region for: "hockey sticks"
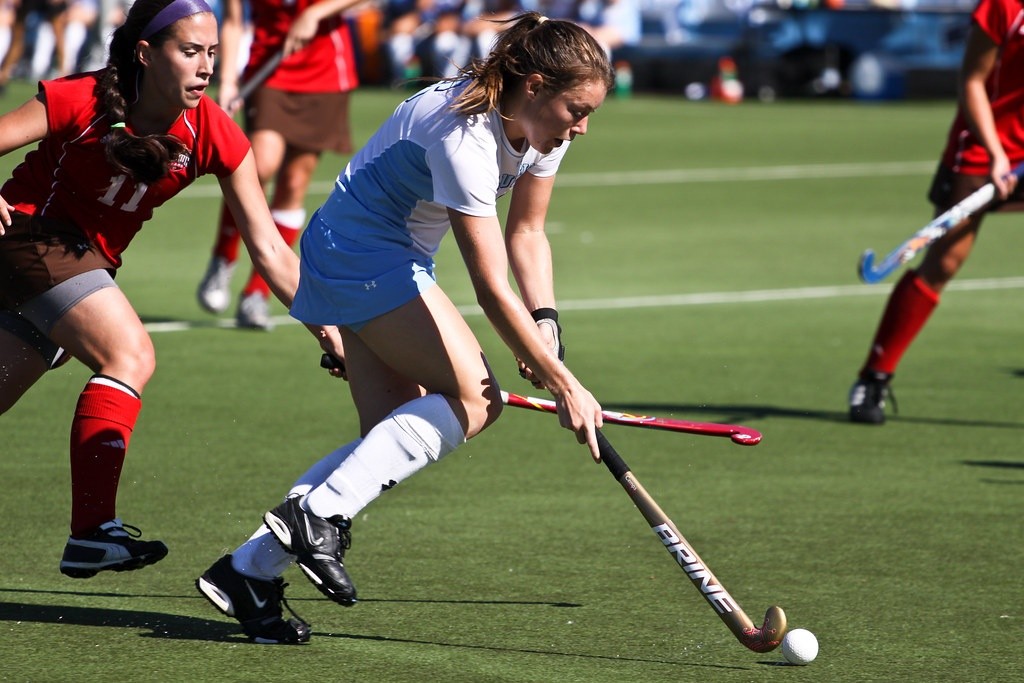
[854,162,1024,287]
[225,50,286,111]
[593,427,789,656]
[317,351,765,448]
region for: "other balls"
[779,627,820,666]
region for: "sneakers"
[193,555,309,644]
[848,368,899,425]
[59,519,167,578]
[261,494,358,607]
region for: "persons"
[197,2,366,331]
[195,11,616,647]
[0,1,346,581]
[0,0,905,106]
[850,0,1023,427]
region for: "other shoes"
[198,252,229,312]
[237,292,270,329]
